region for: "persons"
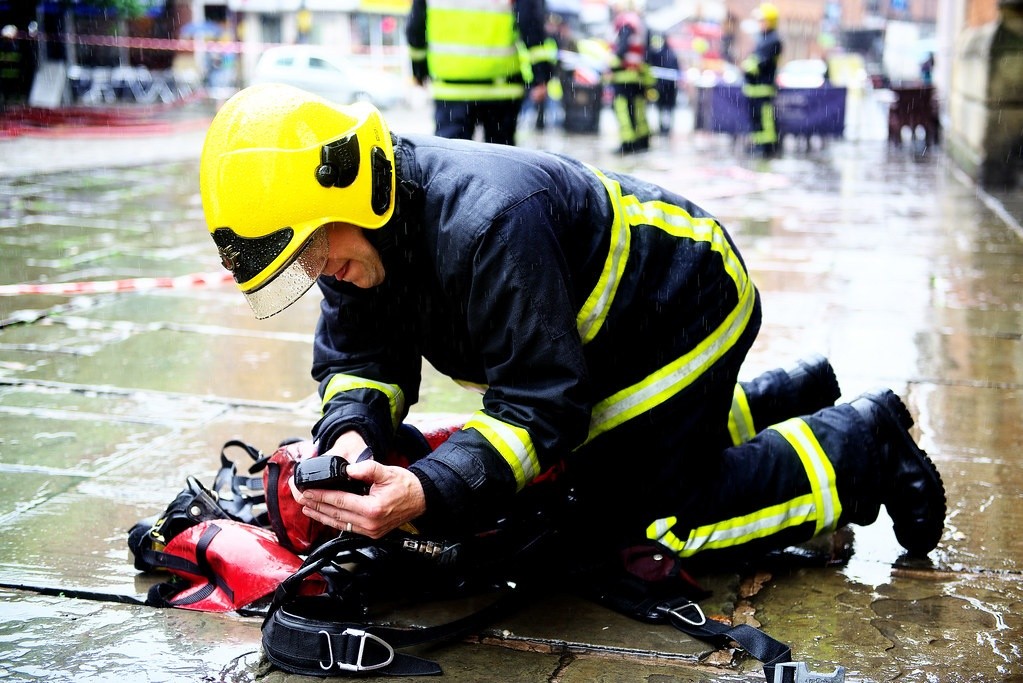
[406,0,556,147]
[531,1,680,151]
[201,83,947,581]
[743,6,782,155]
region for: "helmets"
[198,80,396,295]
[753,1,780,31]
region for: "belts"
[262,603,482,675]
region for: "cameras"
[292,455,372,498]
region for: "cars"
[696,57,848,136]
[250,47,405,112]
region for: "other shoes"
[617,137,648,153]
[746,141,779,157]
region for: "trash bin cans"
[565,81,603,136]
[693,86,765,134]
[775,84,848,151]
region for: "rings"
[347,523,352,532]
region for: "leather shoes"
[783,352,844,415]
[851,389,946,556]
